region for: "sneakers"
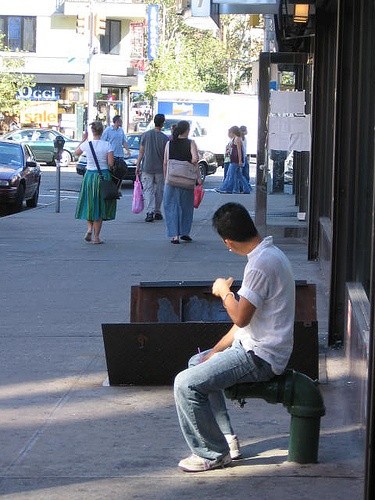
[223,435,242,460]
[177,452,231,472]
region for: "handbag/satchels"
[88,141,118,200]
[132,175,144,214]
[167,140,197,190]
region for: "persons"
[99,116,129,198]
[217,126,250,194]
[174,202,293,471]
[75,122,117,244]
[163,121,198,243]
[136,114,169,222]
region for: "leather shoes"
[144,213,154,222]
[154,213,163,220]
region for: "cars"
[0,139,41,212]
[2,128,80,166]
[76,133,217,186]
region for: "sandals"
[84,232,92,242]
[170,238,180,245]
[180,235,191,242]
[92,241,104,245]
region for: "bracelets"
[222,293,235,309]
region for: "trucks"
[146,90,258,154]
